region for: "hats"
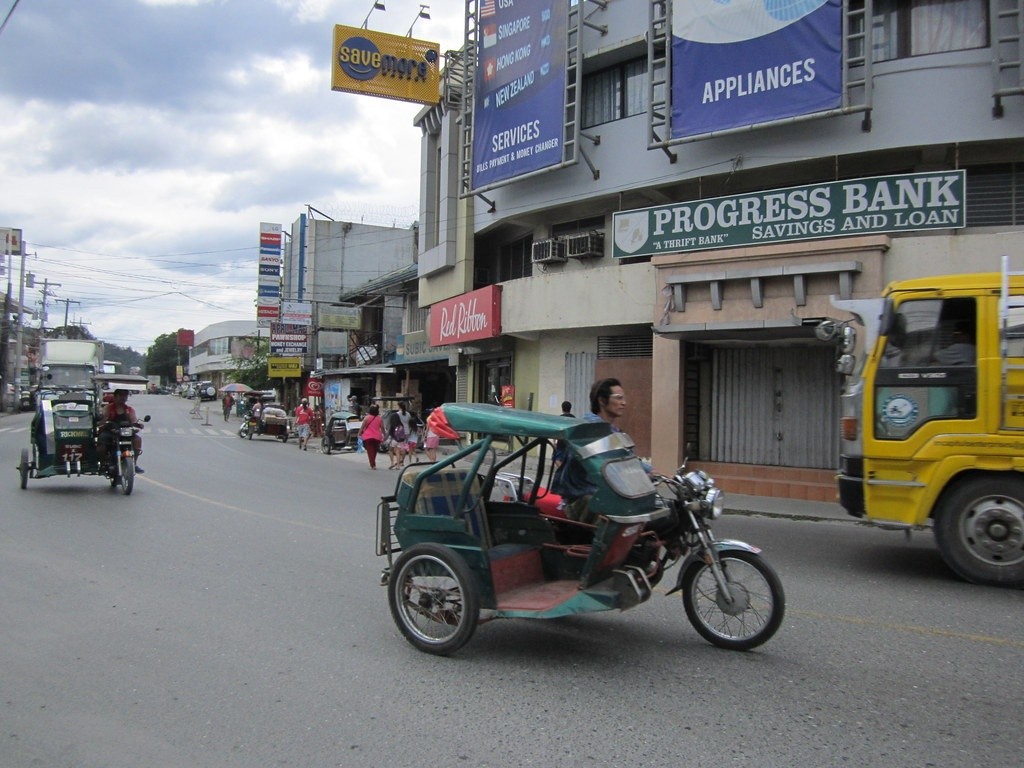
[302,398,308,403]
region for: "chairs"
[403,469,544,594]
[333,426,346,441]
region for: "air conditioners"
[567,231,603,259]
[532,239,567,263]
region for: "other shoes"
[135,465,145,474]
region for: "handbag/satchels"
[394,424,406,441]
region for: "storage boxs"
[264,413,286,425]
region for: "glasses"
[606,393,626,402]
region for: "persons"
[405,418,420,463]
[223,391,234,421]
[396,401,411,465]
[251,399,263,419]
[96,388,144,473]
[425,426,439,462]
[551,376,662,526]
[923,321,976,366]
[294,397,314,451]
[553,401,575,467]
[349,396,361,416]
[359,404,386,470]
[383,412,403,470]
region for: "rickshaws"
[15,387,152,496]
[372,394,429,455]
[237,389,290,444]
[320,412,364,455]
[370,397,786,657]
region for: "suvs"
[193,380,217,402]
[173,381,197,399]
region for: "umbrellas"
[219,383,252,392]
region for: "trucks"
[31,336,105,420]
[797,255,1024,589]
